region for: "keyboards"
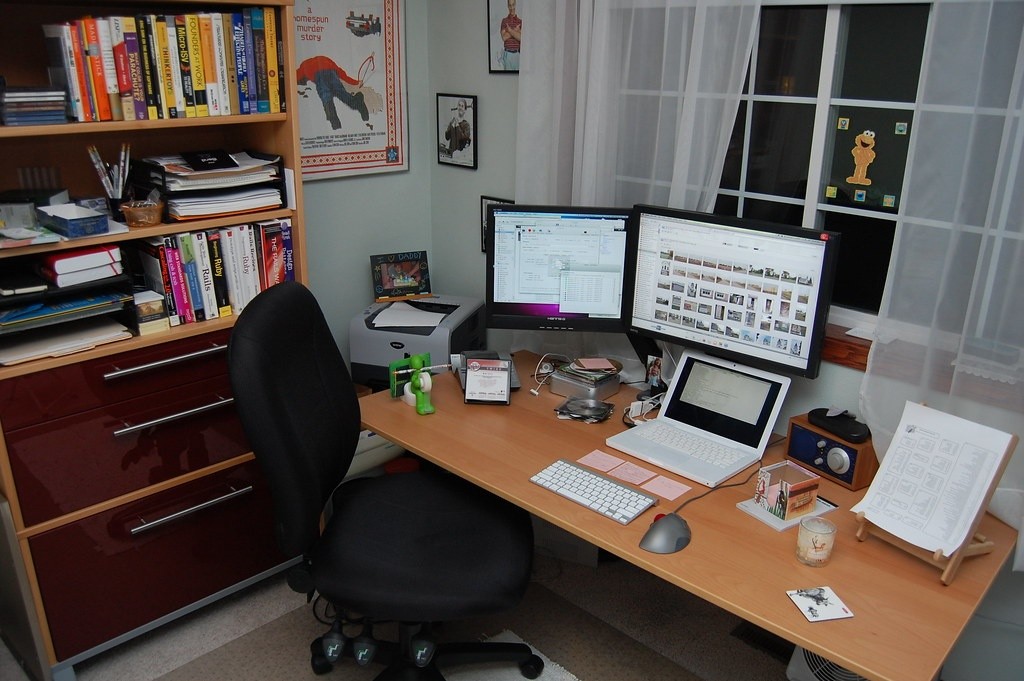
[528,458,659,525]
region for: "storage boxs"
[344,380,406,479]
[755,460,820,521]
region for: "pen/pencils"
[86,141,135,199]
[392,364,454,375]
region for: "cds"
[567,400,607,414]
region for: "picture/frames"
[481,195,516,254]
[486,0,522,74]
[370,250,431,299]
[436,92,478,170]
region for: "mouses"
[639,512,692,553]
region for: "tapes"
[418,373,432,393]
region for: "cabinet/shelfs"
[0,0,308,681]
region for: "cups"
[107,195,131,222]
[795,516,838,567]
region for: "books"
[140,218,295,327]
[0,271,47,295]
[41,244,122,287]
[736,495,839,532]
[0,7,288,125]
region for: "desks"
[357,350,1019,681]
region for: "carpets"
[434,629,581,681]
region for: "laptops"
[606,347,792,488]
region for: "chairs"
[228,280,544,681]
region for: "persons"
[500,0,522,70]
[444,99,470,154]
[390,262,403,278]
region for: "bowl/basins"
[119,200,166,227]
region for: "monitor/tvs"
[630,204,843,380]
[484,203,633,334]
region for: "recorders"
[785,412,880,491]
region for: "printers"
[349,293,487,389]
